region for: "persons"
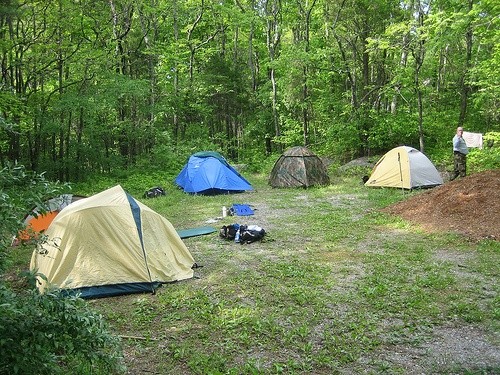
[449,126,468,180]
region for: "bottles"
[222,206,227,217]
[235,229,241,243]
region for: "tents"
[30,185,196,300]
[175,151,254,194]
[12,195,90,248]
[268,147,330,189]
[363,146,444,189]
